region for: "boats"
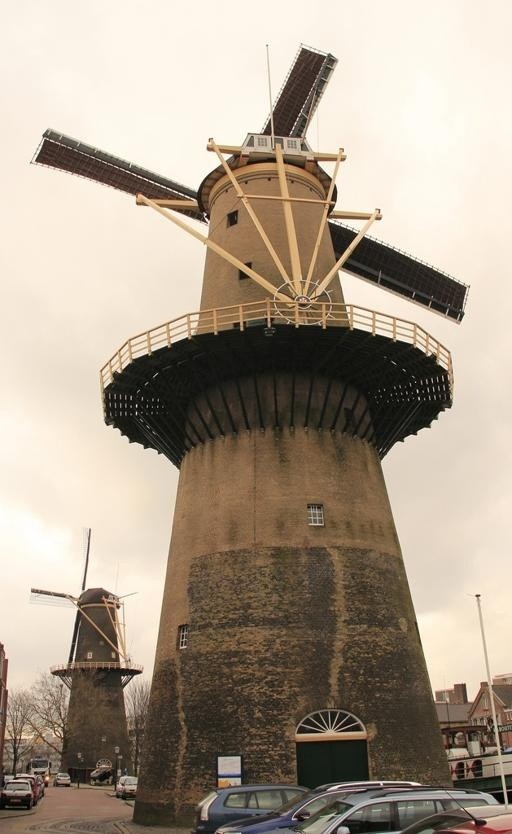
[442,725,512,795]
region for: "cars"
[53,772,71,787]
[0,774,45,810]
[115,775,139,800]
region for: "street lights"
[77,752,82,789]
[114,746,120,788]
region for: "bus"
[26,758,51,787]
